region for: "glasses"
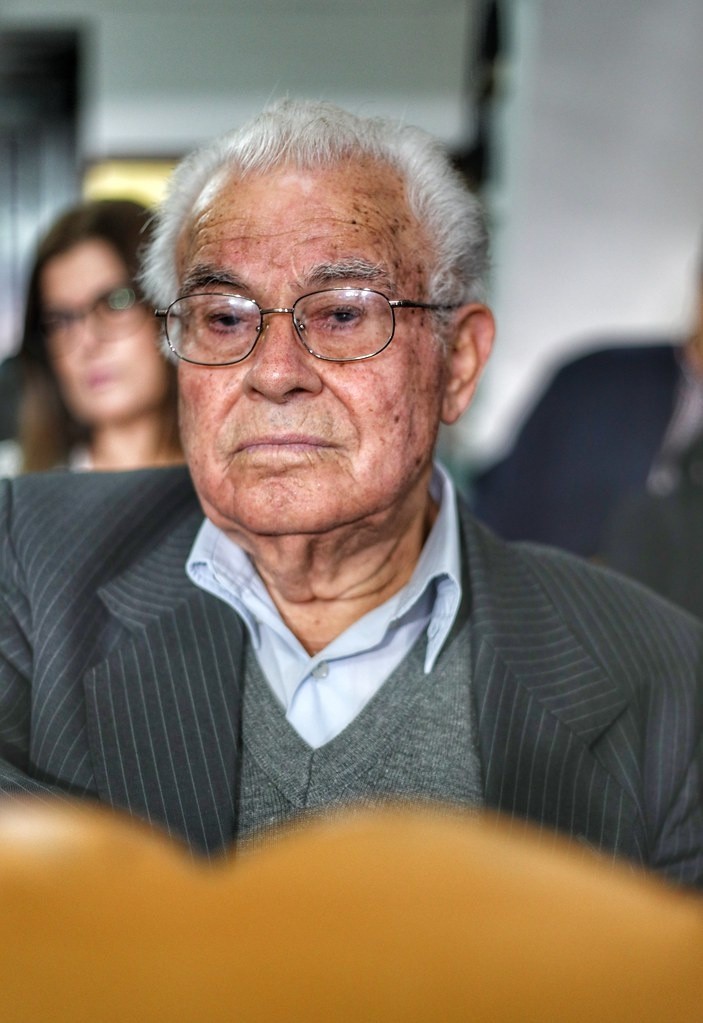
[154,286,461,362]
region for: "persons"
[469,255,702,617]
[0,198,187,476]
[0,90,702,1023]
